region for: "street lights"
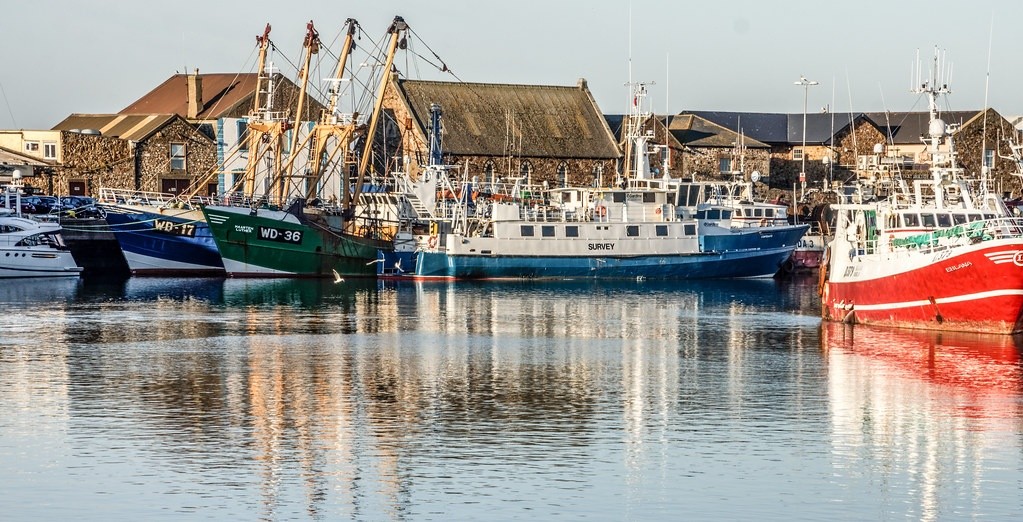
[360,63,382,182]
[794,76,820,203]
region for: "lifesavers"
[357,225,370,237]
[427,236,438,249]
[595,206,607,218]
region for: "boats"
[0,11,813,287]
[815,43,1023,337]
